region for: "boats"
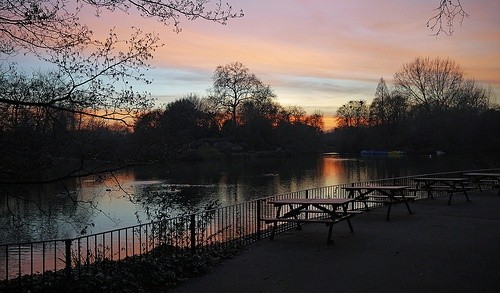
[321,152,340,158]
[360,150,406,157]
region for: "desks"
[341,185,413,221]
[462,172,500,192]
[267,198,356,245]
[410,176,472,205]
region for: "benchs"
[479,179,498,182]
[352,199,398,203]
[258,218,340,223]
[427,185,475,189]
[406,188,456,191]
[364,194,419,199]
[296,209,364,214]
